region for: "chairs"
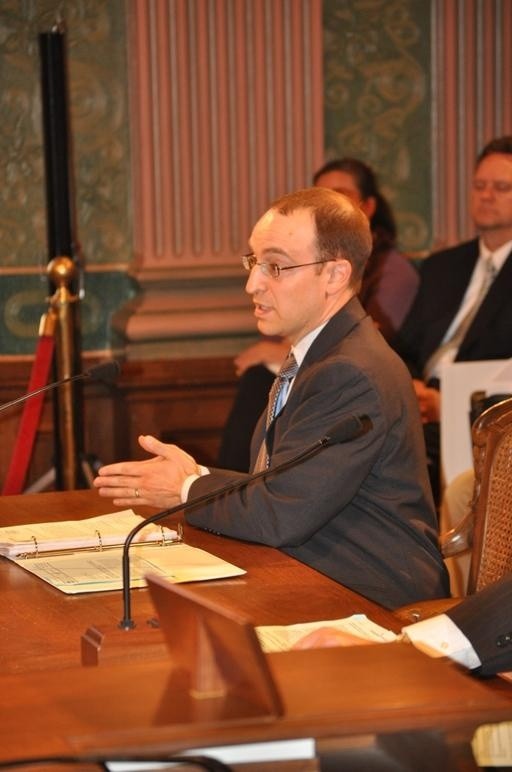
[392,397,512,624]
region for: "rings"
[135,488,139,497]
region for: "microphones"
[0,359,122,413]
[121,411,375,627]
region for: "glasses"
[242,252,337,278]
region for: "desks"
[0,487,512,771]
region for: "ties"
[423,254,498,385]
[266,353,299,469]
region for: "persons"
[392,135,512,498]
[292,565,511,680]
[216,159,422,473]
[94,188,448,611]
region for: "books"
[1,508,247,595]
